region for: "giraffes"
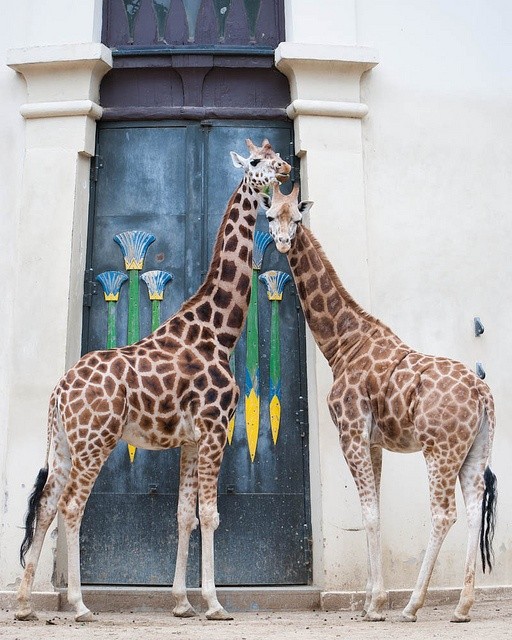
[258,181,497,623]
[14,138,292,622]
